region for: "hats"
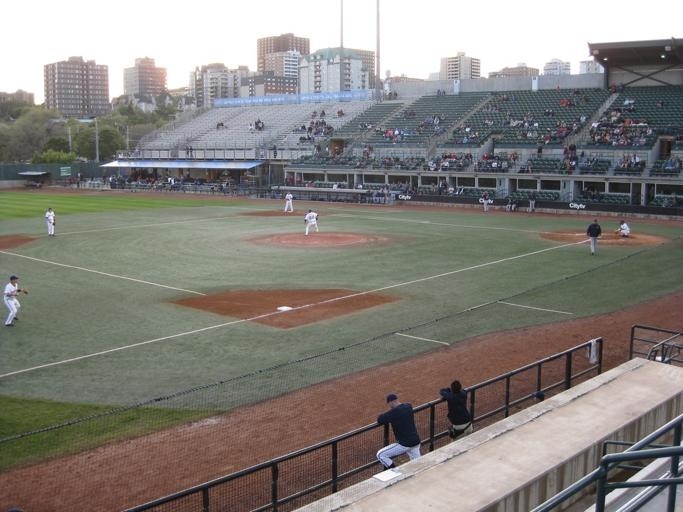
[386,394,397,402]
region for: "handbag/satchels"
[448,427,464,438]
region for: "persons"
[64,146,216,194]
[386,81,683,214]
[216,107,386,205]
[615,220,631,238]
[45,207,57,237]
[2,275,28,327]
[375,392,421,472]
[439,379,475,443]
[586,218,602,256]
[283,191,296,212]
[304,209,319,236]
[531,391,545,404]
